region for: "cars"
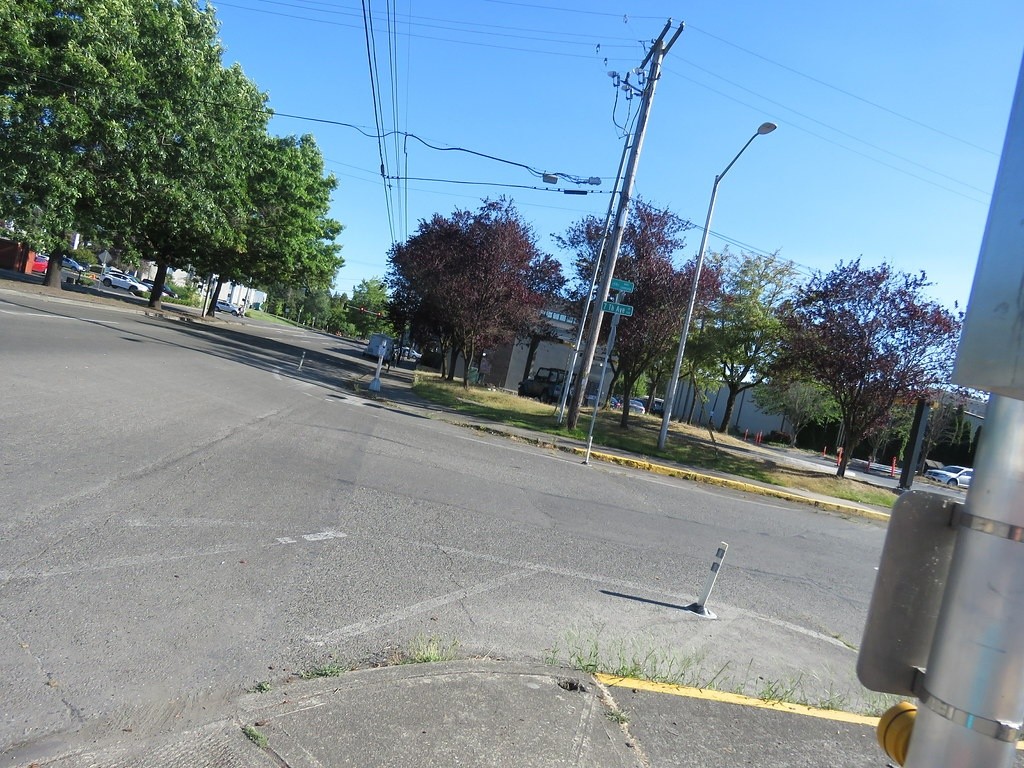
[215,301,240,317]
[608,396,666,413]
[61,257,84,272]
[31,256,49,274]
[398,347,423,360]
[99,269,179,300]
[923,465,974,488]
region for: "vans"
[362,333,396,363]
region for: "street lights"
[654,122,779,449]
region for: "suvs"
[518,368,589,407]
